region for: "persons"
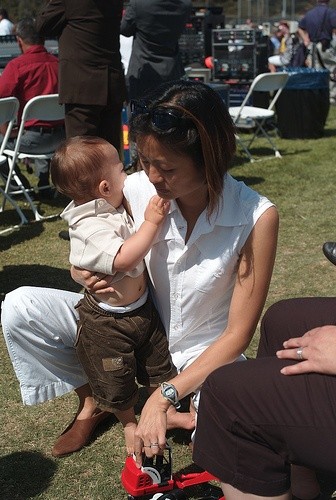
[297,0,336,104]
[0,18,68,202]
[1,81,280,458]
[192,297,336,500]
[0,9,17,35]
[268,23,293,73]
[120,0,191,161]
[49,135,196,455]
[35,0,128,160]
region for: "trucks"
[121,440,226,500]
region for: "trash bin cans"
[274,68,330,140]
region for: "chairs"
[228,72,288,163]
[0,97,28,235]
[0,94,65,222]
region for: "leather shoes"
[322,241,336,267]
[52,411,114,456]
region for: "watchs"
[160,382,181,409]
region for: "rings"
[297,347,303,359]
[151,443,158,446]
[143,446,151,447]
[95,273,101,280]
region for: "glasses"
[131,98,185,130]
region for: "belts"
[22,124,62,134]
